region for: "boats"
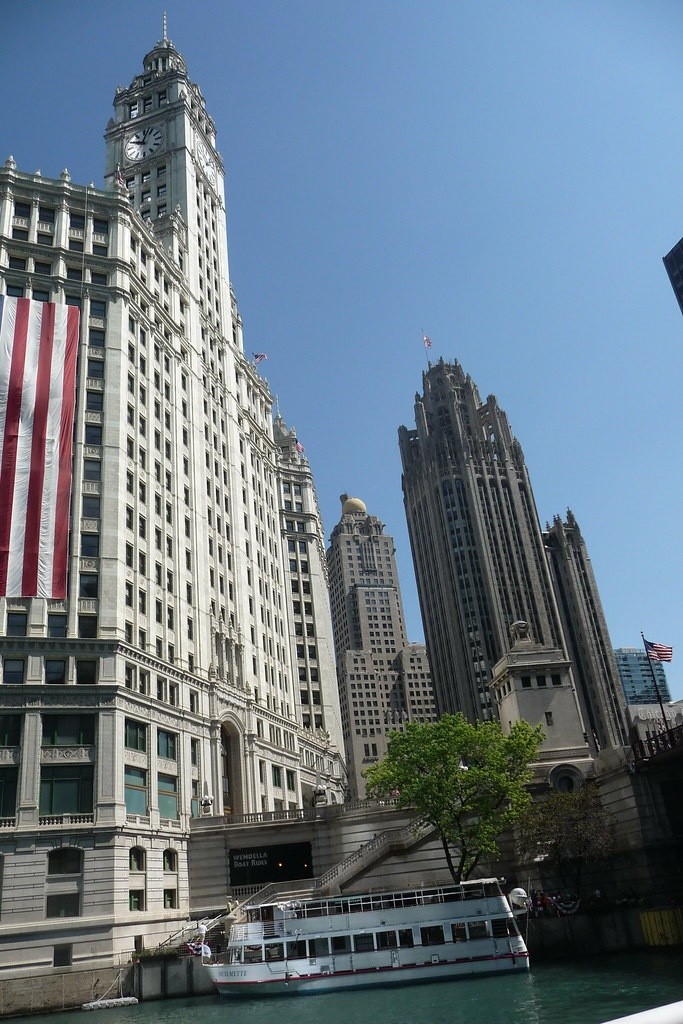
[196,876,533,998]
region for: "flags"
[644,638,673,664]
[0,297,85,604]
[295,438,305,454]
[424,334,432,350]
[253,352,268,365]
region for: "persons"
[226,899,232,914]
[528,886,642,919]
[234,897,239,908]
[392,785,400,796]
[198,922,210,944]
